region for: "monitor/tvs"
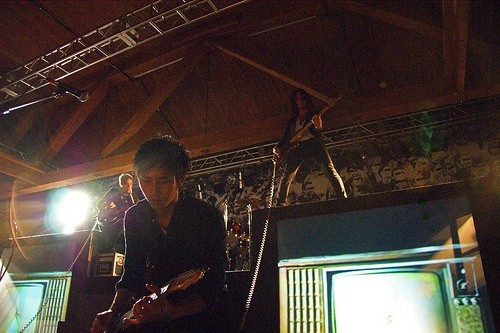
[245,177,499,332]
[9,271,73,333]
[276,244,484,333]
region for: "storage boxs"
[95,252,125,277]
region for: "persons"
[88,134,238,333]
[271,87,350,208]
[96,173,137,253]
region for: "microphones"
[96,187,114,208]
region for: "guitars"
[96,198,135,227]
[99,264,216,333]
[273,94,342,164]
